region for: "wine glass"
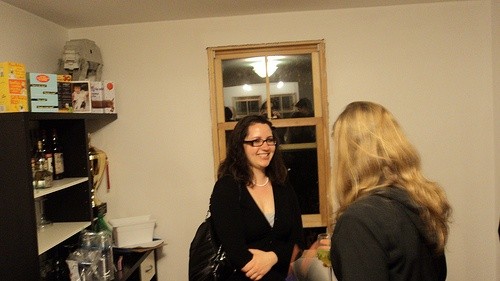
[316,233,333,281]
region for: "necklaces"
[252,176,269,187]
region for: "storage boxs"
[0,61,28,113]
[113,221,155,246]
[90,80,116,113]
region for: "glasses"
[243,138,277,147]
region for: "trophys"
[88,132,110,220]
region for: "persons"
[224,97,319,215]
[72,83,89,110]
[207,115,305,281]
[189,217,315,281]
[316,101,453,281]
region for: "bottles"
[36,140,47,181]
[46,138,53,180]
[94,217,113,245]
[49,134,66,180]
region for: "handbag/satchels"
[188,171,242,281]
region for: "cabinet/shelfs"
[0,112,158,281]
